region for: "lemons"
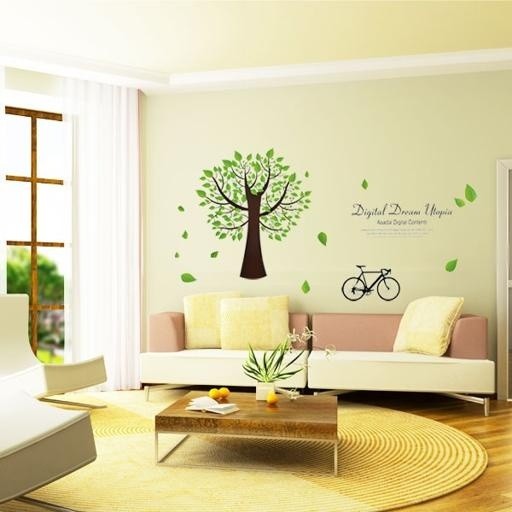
[267,392,278,405]
[208,386,230,400]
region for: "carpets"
[14,390,488,512]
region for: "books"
[184,396,241,416]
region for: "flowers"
[243,326,338,400]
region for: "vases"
[256,382,278,401]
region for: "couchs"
[308,313,496,416]
[140,312,310,401]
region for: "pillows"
[185,292,251,350]
[220,295,293,350]
[393,296,464,356]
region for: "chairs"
[1,293,107,408]
[0,387,97,504]
